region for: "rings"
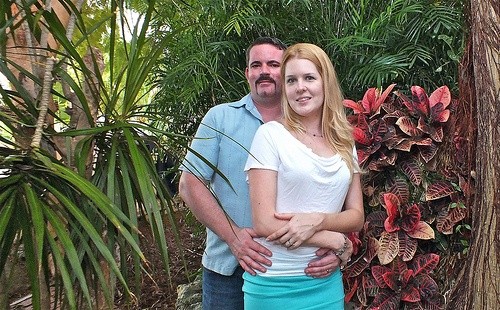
[287,239,292,246]
[327,270,330,274]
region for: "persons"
[242,43,364,310]
[178,37,341,310]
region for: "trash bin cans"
[156,162,176,199]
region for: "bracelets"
[335,233,350,257]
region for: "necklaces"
[310,131,324,138]
